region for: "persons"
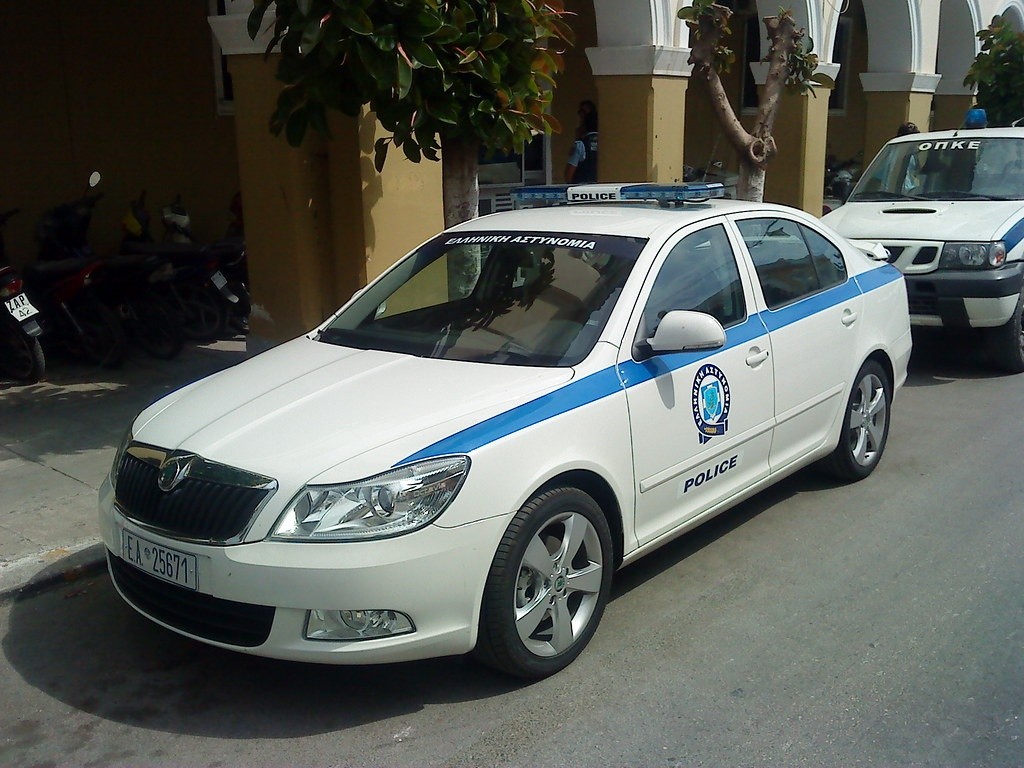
[563,101,599,184]
[885,122,923,196]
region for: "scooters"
[0,171,253,384]
[823,151,861,203]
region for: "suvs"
[818,110,1024,375]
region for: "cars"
[98,182,914,682]
